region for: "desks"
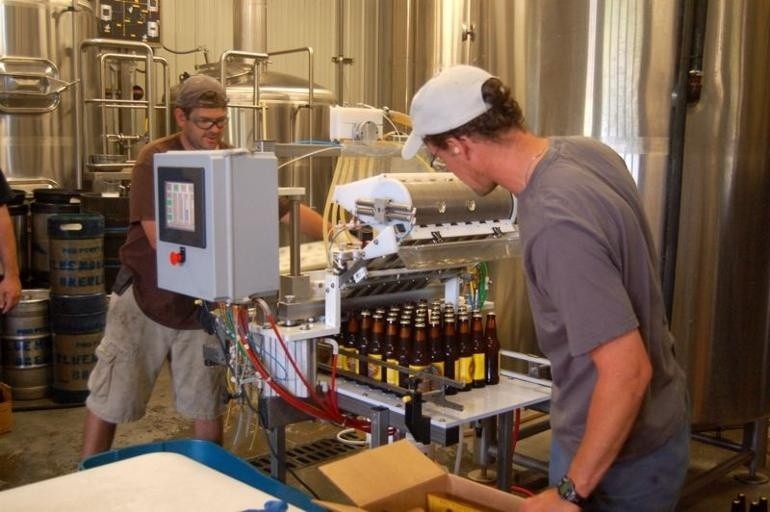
[0,449,310,512]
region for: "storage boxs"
[311,439,526,512]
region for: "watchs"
[556,475,586,508]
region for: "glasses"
[429,139,451,170]
[185,116,229,130]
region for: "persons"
[81,74,334,461]
[0,168,22,317]
[400,64,692,511]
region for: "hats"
[401,65,502,159]
[170,75,229,108]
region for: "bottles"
[319,296,501,403]
